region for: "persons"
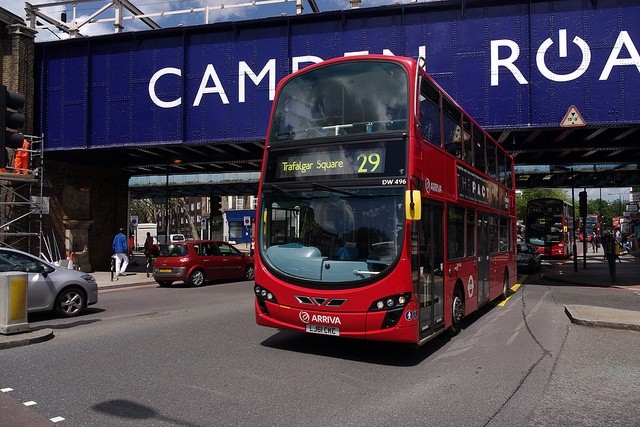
[603,230,618,282]
[113,228,130,277]
[590,232,600,252]
[144,232,153,257]
[14,132,30,176]
[620,234,627,246]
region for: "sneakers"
[116,273,121,276]
[119,271,126,276]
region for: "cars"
[0,247,97,316]
[517,244,541,270]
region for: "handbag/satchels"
[596,242,600,248]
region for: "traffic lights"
[0,85,25,168]
[210,196,222,216]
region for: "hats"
[120,228,125,230]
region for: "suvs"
[153,240,254,286]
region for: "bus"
[579,214,598,241]
[525,198,574,257]
[254,54,518,346]
